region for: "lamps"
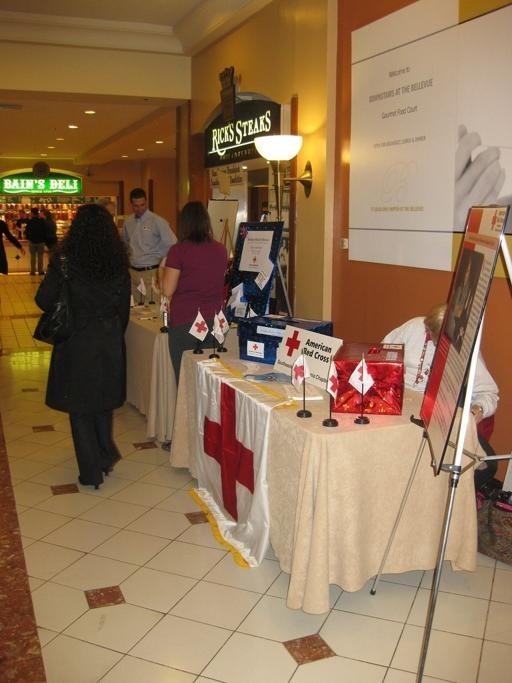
[253,134,315,223]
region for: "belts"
[130,264,158,271]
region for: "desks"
[172,347,478,614]
[129,304,238,443]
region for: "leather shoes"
[78,455,115,489]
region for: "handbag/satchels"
[32,302,74,345]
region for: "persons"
[33,203,131,488]
[157,201,227,451]
[122,188,177,305]
[16,207,59,275]
[378,303,500,494]
[454,125,512,232]
[0,216,26,274]
